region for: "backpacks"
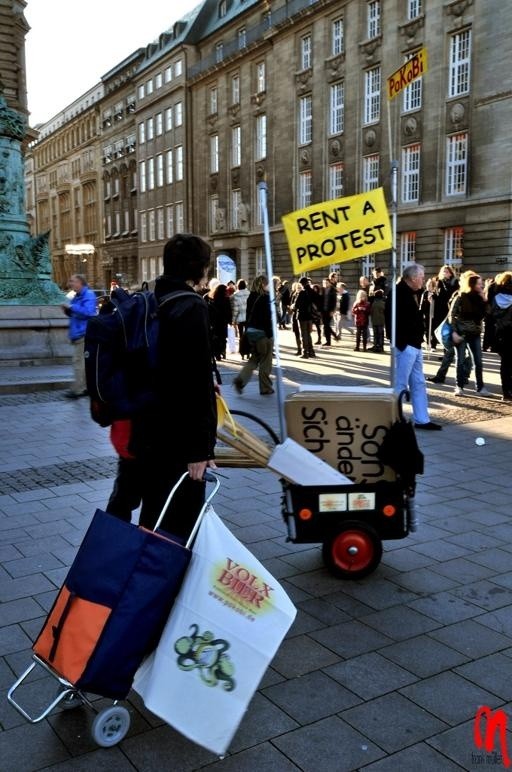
[84,280,205,427]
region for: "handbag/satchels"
[434,317,455,350]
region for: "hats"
[227,280,235,286]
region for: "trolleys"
[216,159,410,581]
[6,467,220,750]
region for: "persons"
[105,234,218,540]
[61,273,97,398]
[196,263,512,431]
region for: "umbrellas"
[376,389,424,475]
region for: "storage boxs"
[284,388,399,485]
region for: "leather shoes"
[426,376,444,383]
[295,348,315,358]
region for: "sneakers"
[60,389,88,400]
[414,422,442,430]
[315,336,340,345]
[454,386,463,396]
[260,389,274,395]
[242,355,250,360]
[475,387,492,396]
[354,345,383,352]
[233,377,244,394]
[216,355,226,360]
